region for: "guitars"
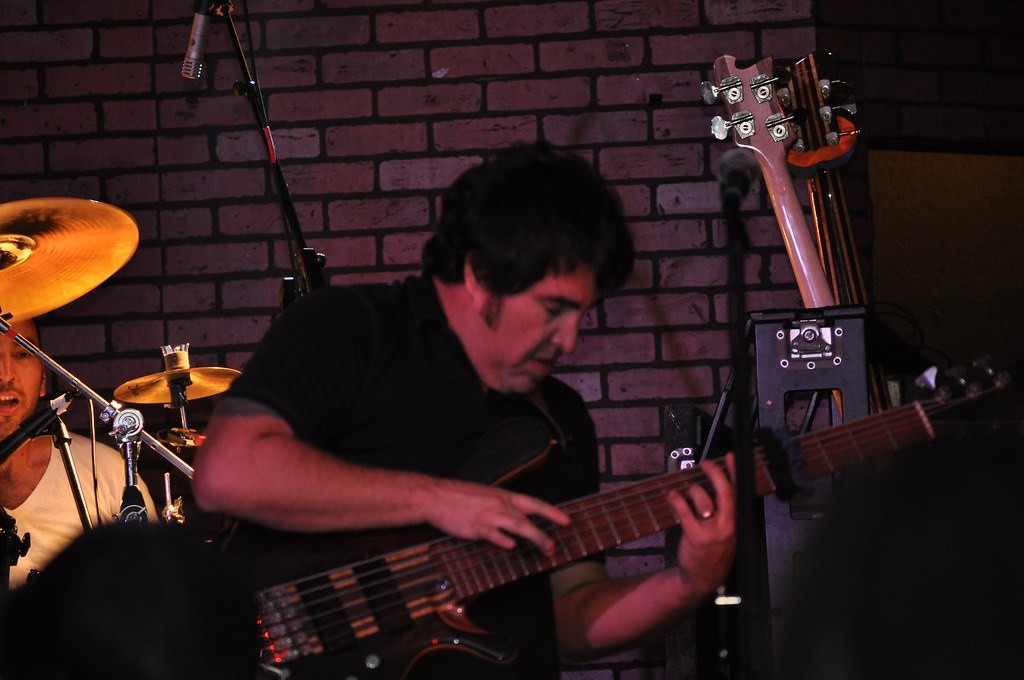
[208,379,1024,680]
[699,49,864,309]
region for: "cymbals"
[0,192,145,333]
[112,364,247,409]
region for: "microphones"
[0,391,75,466]
[717,149,759,203]
[181,0,214,80]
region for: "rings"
[701,511,712,520]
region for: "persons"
[191,137,738,680]
[0,316,163,593]
